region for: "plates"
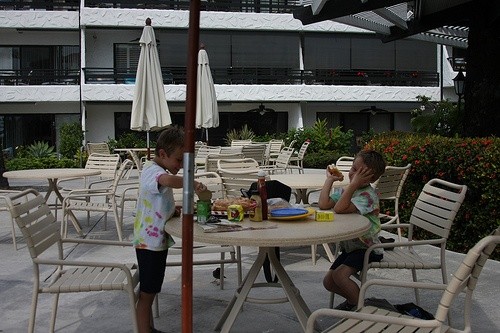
[270,208,308,216]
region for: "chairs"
[0,139,500,333]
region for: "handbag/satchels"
[240,180,292,203]
[395,303,434,320]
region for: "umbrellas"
[130,25,172,160]
[196,49,219,141]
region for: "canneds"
[196,200,211,224]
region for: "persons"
[320,151,386,312]
[134,129,207,333]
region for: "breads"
[328,164,344,180]
[212,197,249,211]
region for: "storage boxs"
[315,210,334,222]
[197,200,211,223]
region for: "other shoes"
[334,299,358,311]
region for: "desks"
[113,148,156,152]
[165,204,372,333]
[3,169,101,233]
[270,174,349,263]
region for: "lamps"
[452,69,466,95]
[259,111,265,115]
[371,110,376,115]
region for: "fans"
[360,106,386,111]
[247,102,275,112]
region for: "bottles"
[249,190,262,222]
[258,171,268,221]
